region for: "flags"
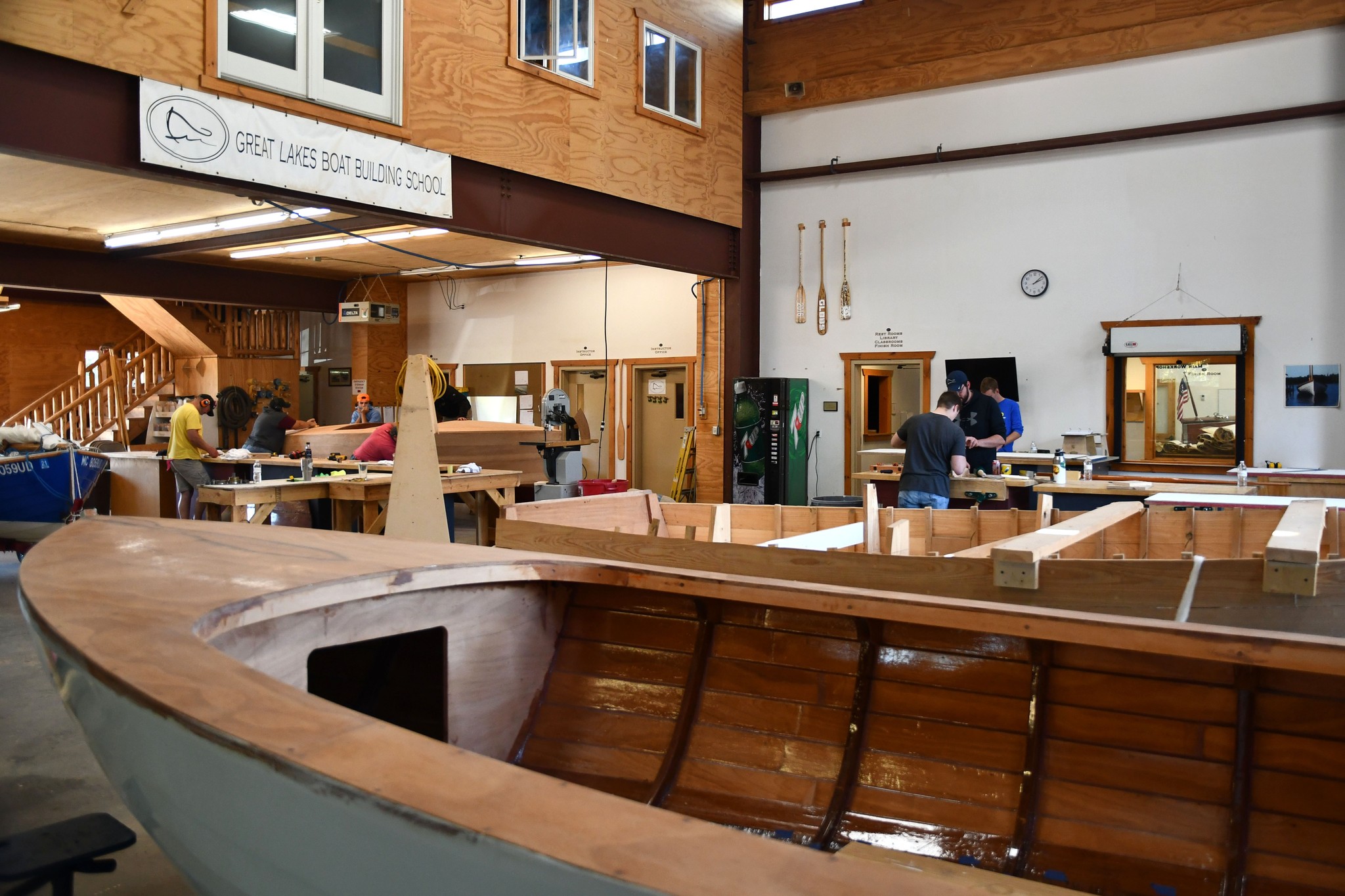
[1176,376,1189,423]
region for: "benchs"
[990,501,1145,591]
[755,521,865,552]
[1260,499,1327,598]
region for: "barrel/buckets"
[810,495,863,508]
[578,479,629,497]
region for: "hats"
[197,394,215,416]
[357,393,370,402]
[945,370,968,391]
[270,397,291,408]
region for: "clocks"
[1021,270,1048,297]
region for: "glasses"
[956,383,966,393]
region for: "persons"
[430,376,473,423]
[167,394,218,520]
[350,393,382,424]
[350,422,399,462]
[891,370,1024,509]
[242,398,319,456]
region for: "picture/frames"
[328,367,352,387]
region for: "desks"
[99,450,523,548]
[850,452,1345,511]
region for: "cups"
[1001,464,1011,475]
[447,465,453,474]
[301,458,313,481]
[358,464,368,479]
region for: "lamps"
[398,253,602,275]
[104,204,331,249]
[229,227,449,259]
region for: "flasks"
[1052,448,1066,484]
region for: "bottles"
[305,442,312,459]
[1083,457,1093,482]
[253,459,262,484]
[1030,440,1037,454]
[734,381,765,480]
[1237,460,1247,488]
